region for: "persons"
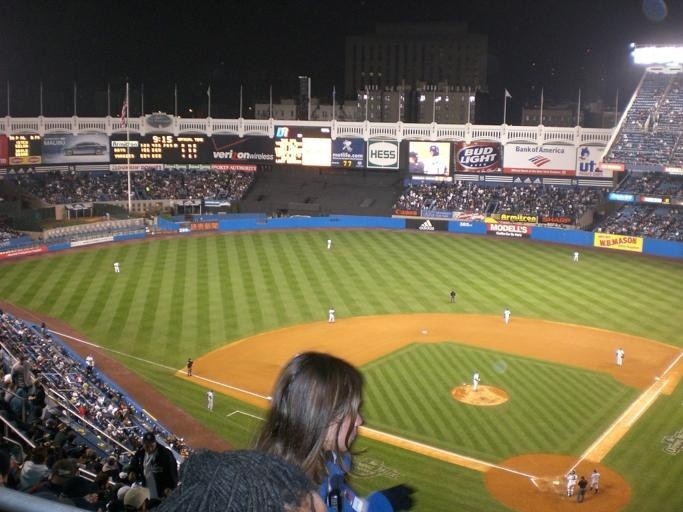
[206,389,215,413]
[503,308,510,324]
[450,291,455,303]
[0,308,196,511]
[247,352,415,511]
[577,476,588,503]
[157,447,331,511]
[473,369,482,392]
[393,180,599,227]
[572,250,579,264]
[566,470,577,496]
[588,468,600,494]
[616,347,624,366]
[9,170,258,208]
[327,239,331,249]
[595,174,683,242]
[113,261,119,272]
[327,306,336,323]
[608,73,683,169]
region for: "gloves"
[380,483,416,511]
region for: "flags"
[120,94,128,129]
[505,89,511,97]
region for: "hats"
[123,486,150,509]
[117,486,130,499]
[143,432,156,444]
